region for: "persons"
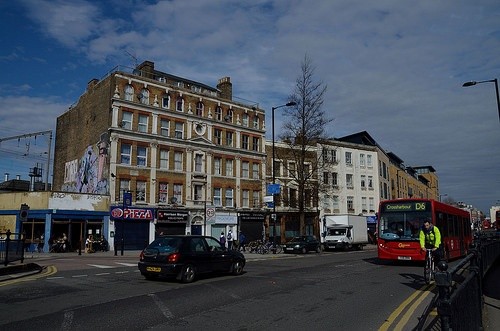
[420,221,442,275]
[39,233,108,253]
[396,225,404,236]
[220,230,246,253]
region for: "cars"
[473,234,487,243]
[283,235,322,254]
[138,236,246,284]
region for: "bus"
[378,198,472,261]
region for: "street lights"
[462,78,500,122]
[270,102,296,254]
[396,169,412,198]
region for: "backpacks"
[227,232,232,240]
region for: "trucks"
[323,214,367,251]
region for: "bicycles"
[256,239,282,255]
[419,249,439,284]
